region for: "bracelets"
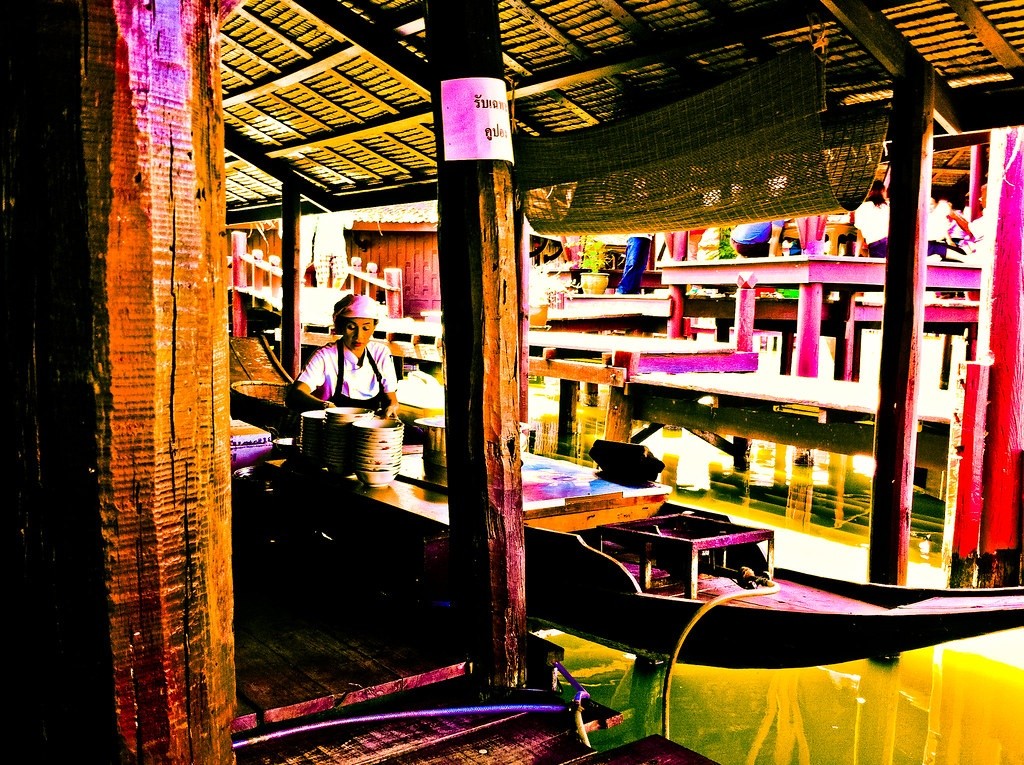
[323,401,334,409]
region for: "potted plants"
[565,234,610,296]
[528,258,583,327]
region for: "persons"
[730,220,784,258]
[615,233,655,295]
[284,295,399,421]
[854,178,988,301]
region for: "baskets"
[230,381,305,438]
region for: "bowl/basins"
[273,438,294,452]
[300,408,405,488]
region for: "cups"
[580,273,610,295]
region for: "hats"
[333,294,380,334]
